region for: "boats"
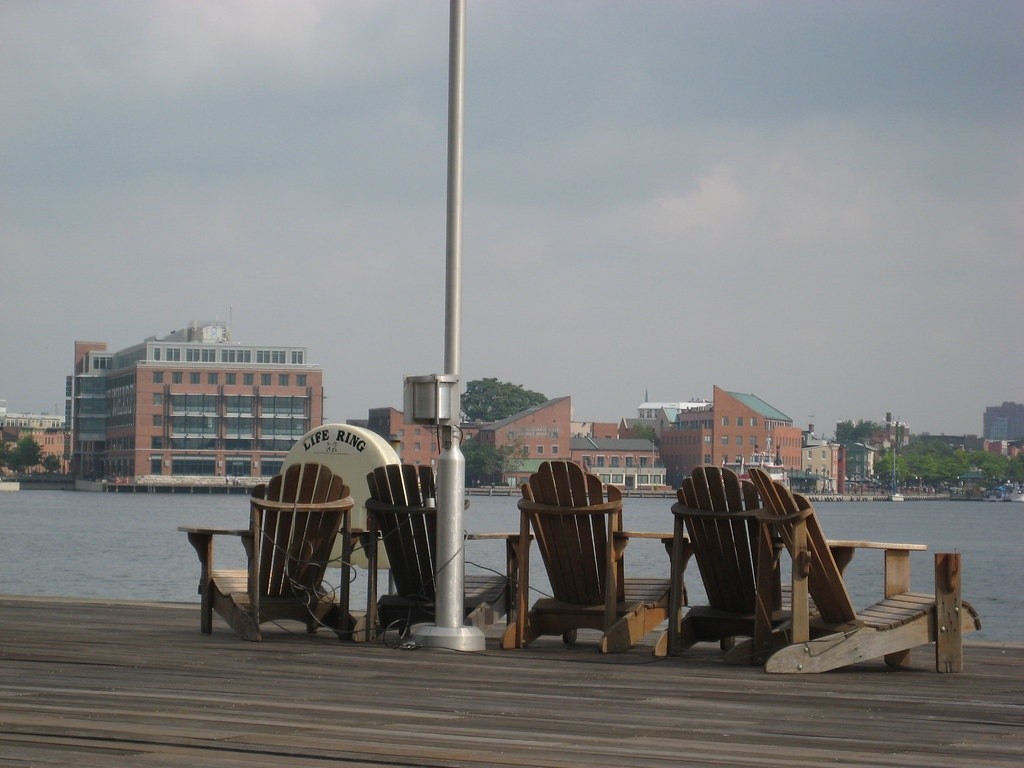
[891,494,904,502]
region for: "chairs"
[651,465,786,671]
[500,460,694,654]
[177,463,357,642]
[351,464,535,642]
[723,467,984,674]
